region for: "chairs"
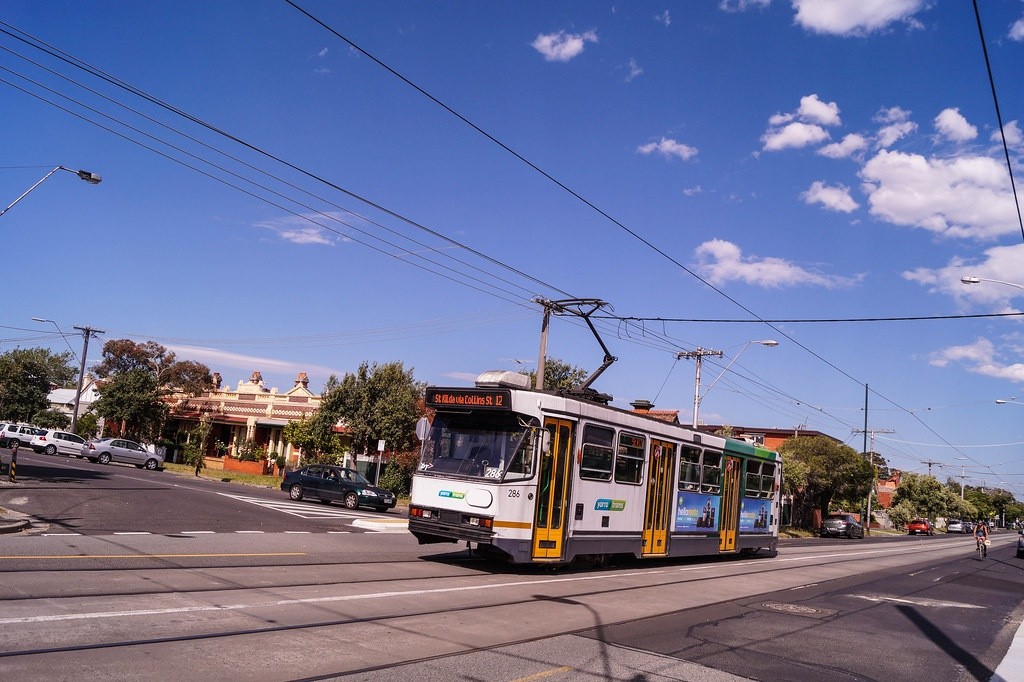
[469,447,491,462]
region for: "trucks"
[1006,518,1024,529]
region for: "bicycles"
[975,535,987,560]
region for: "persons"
[327,470,335,480]
[974,520,988,556]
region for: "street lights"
[31,317,83,435]
[693,340,779,431]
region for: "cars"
[280,465,398,512]
[964,522,974,533]
[1017,528,1024,557]
[81,438,163,471]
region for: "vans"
[29,429,86,458]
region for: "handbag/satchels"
[984,540,991,545]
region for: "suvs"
[948,520,965,533]
[821,514,864,539]
[0,422,38,449]
[909,519,933,536]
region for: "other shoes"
[976,548,979,550]
[984,555,987,559]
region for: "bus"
[407,369,785,572]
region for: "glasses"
[978,523,981,524]
